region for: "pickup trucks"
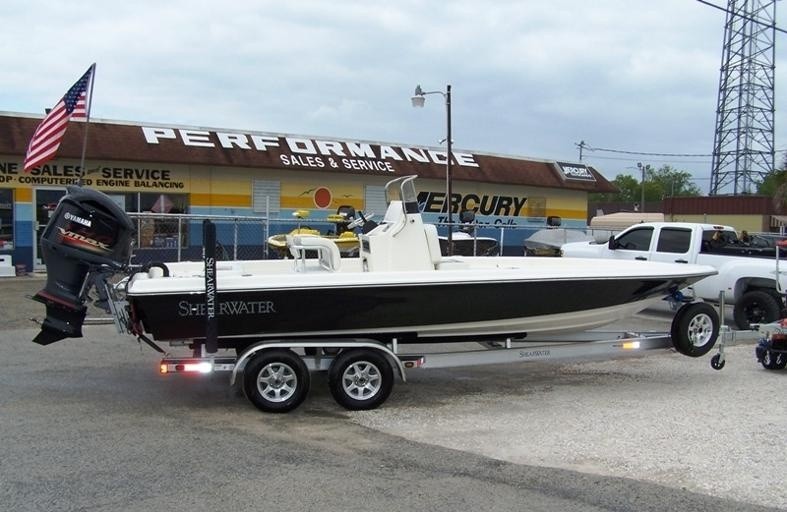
[559,222,786,331]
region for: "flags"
[22,63,94,173]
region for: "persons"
[708,230,728,254]
[740,230,749,247]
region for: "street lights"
[410,83,455,256]
[637,162,650,212]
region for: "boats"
[521,215,597,257]
[437,210,499,256]
[266,206,367,258]
[29,174,717,337]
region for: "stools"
[291,234,341,272]
